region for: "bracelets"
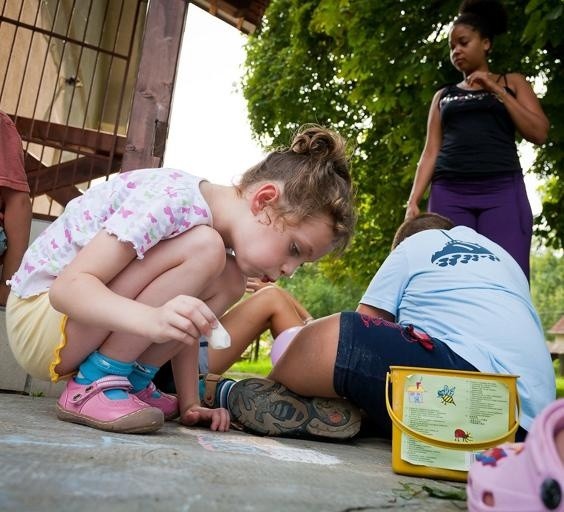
[494,88,507,103]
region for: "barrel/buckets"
[383,365,521,481]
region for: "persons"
[1,110,34,309]
[217,214,559,444]
[4,123,355,434]
[199,270,317,375]
[404,13,552,293]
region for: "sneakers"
[54,374,180,434]
[224,377,364,440]
